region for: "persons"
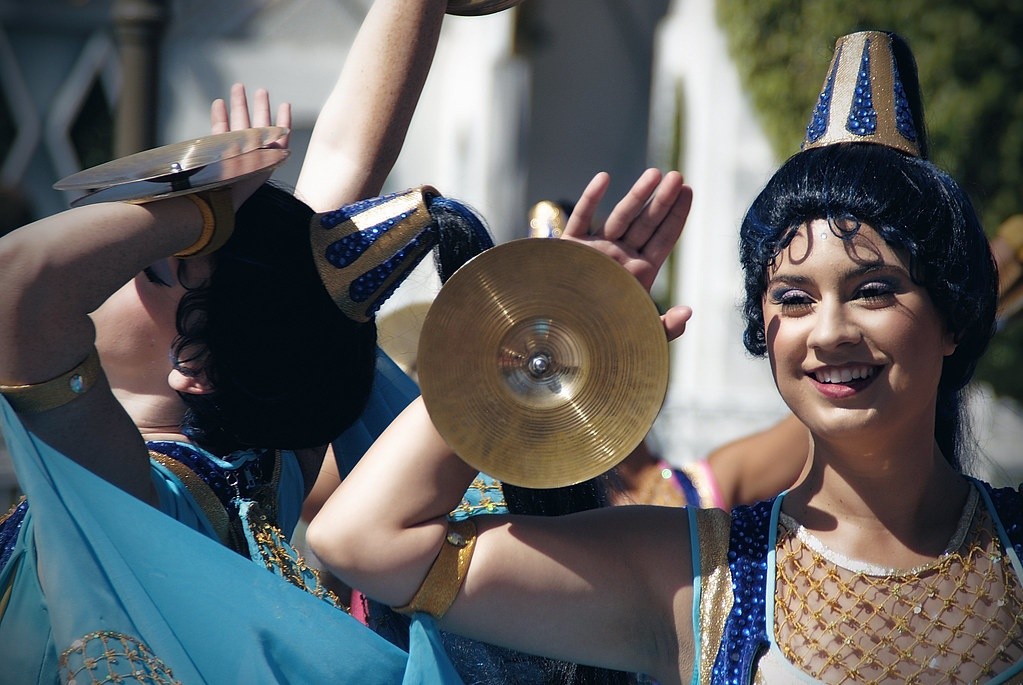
[0,0,808,683]
[304,143,1023,685]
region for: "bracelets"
[173,193,216,257]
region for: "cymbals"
[51,123,291,212]
[415,231,673,494]
